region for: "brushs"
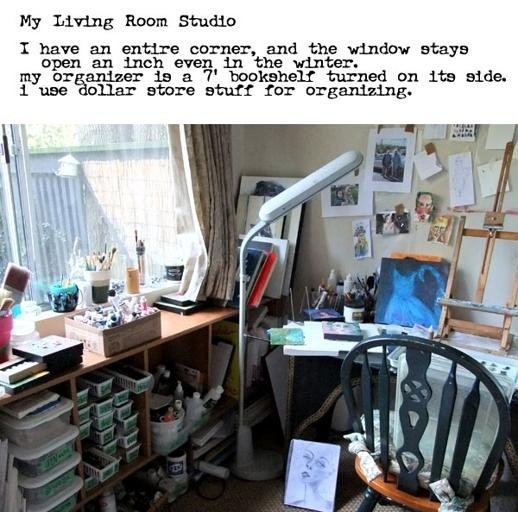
[0,261,32,316]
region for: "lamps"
[232,147,364,482]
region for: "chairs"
[339,335,512,511]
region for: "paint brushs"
[134,231,144,283]
[84,242,116,271]
[357,275,374,302]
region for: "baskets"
[77,362,153,483]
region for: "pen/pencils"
[311,284,341,313]
[60,275,72,287]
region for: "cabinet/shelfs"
[0,289,287,512]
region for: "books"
[323,321,364,342]
[209,232,289,308]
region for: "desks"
[281,316,517,489]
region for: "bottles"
[154,362,200,422]
[336,281,344,296]
[343,272,356,295]
[126,267,140,294]
[326,268,336,291]
[200,384,228,412]
[100,489,116,512]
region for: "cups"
[48,267,111,312]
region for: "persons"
[344,186,356,205]
[383,215,399,235]
[334,190,344,205]
[392,149,400,178]
[382,150,392,177]
[396,215,407,233]
[317,310,338,319]
[354,225,368,257]
